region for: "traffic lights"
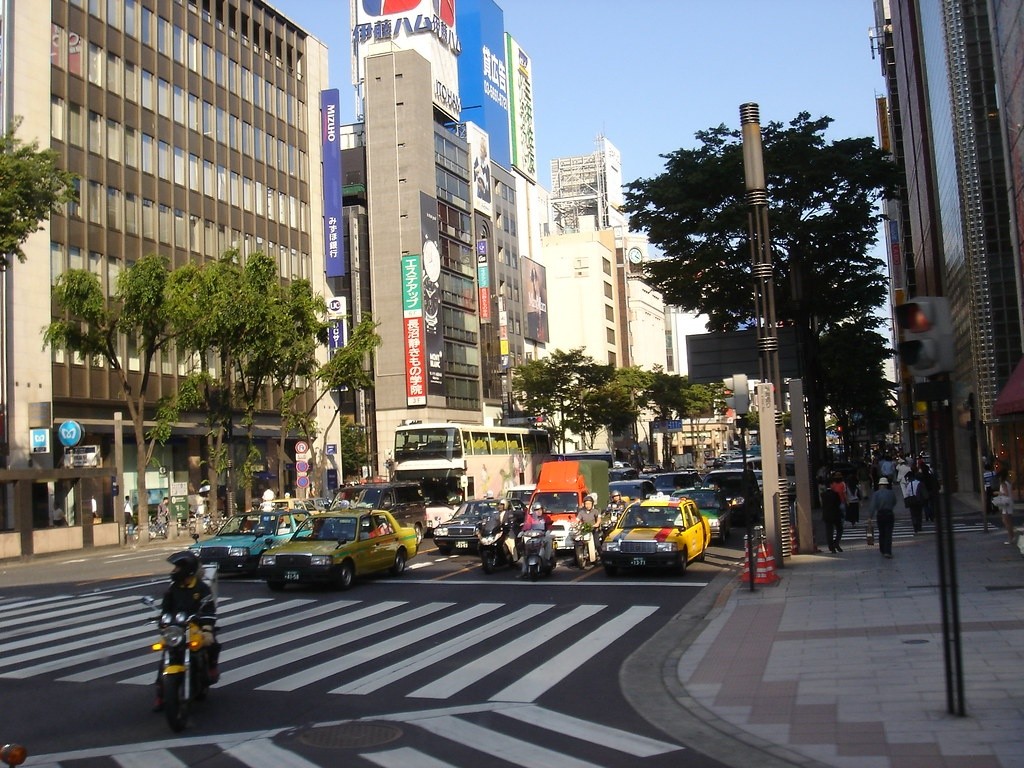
[894,293,956,377]
[724,373,749,414]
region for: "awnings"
[992,356,1024,416]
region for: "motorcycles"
[568,507,624,568]
[516,510,556,581]
[140,593,218,733]
[474,508,522,573]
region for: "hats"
[878,477,889,484]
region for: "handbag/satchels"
[865,521,874,545]
[904,495,917,508]
[992,492,1010,507]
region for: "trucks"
[519,460,609,552]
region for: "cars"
[609,455,796,498]
[432,500,529,555]
[673,488,730,543]
[599,499,711,575]
[608,480,656,501]
[258,498,331,530]
[188,510,312,578]
[259,507,418,590]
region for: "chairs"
[403,439,519,451]
[277,521,286,535]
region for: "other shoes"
[208,660,219,680]
[830,542,843,554]
[153,696,163,712]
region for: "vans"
[700,468,760,527]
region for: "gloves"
[545,530,550,535]
[517,531,524,538]
[202,625,214,646]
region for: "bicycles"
[133,516,168,542]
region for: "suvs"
[328,482,427,549]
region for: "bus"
[393,424,549,532]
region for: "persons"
[601,490,627,519]
[124,495,134,525]
[993,470,1018,545]
[151,551,221,714]
[867,477,897,559]
[820,478,844,553]
[816,443,940,533]
[494,499,518,566]
[515,502,554,580]
[157,497,169,541]
[983,464,996,513]
[571,497,605,564]
[92,495,99,519]
[52,501,68,527]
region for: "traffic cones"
[743,541,777,584]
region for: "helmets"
[610,490,621,501]
[497,499,509,509]
[583,496,594,503]
[167,551,198,582]
[532,502,543,512]
